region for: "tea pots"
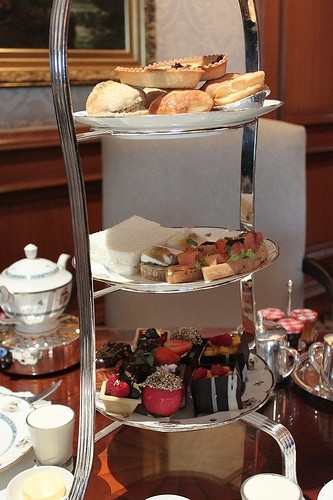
[0,243,73,338]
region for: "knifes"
[25,379,62,405]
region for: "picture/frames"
[0,0,156,90]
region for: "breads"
[86,56,271,114]
[141,231,267,283]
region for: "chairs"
[101,118,307,331]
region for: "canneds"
[256,307,317,360]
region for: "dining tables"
[0,327,333,500]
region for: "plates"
[6,466,74,500]
[0,393,35,472]
[290,347,333,401]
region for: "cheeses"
[21,476,67,500]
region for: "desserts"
[93,325,251,418]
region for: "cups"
[309,334,333,393]
[240,473,303,500]
[254,331,299,382]
[257,308,318,350]
[317,478,333,500]
[25,405,76,465]
